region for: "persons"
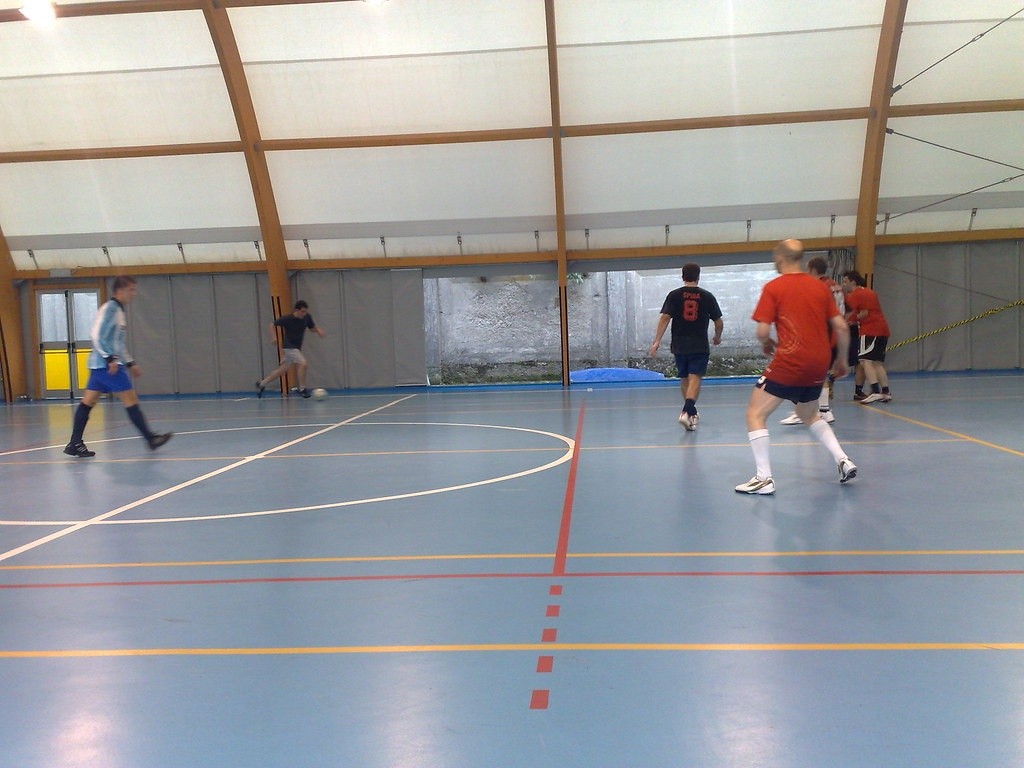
[63,275,172,458]
[648,263,723,431]
[829,270,893,404]
[734,240,858,495]
[255,300,324,398]
[780,256,845,426]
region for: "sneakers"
[854,393,867,400]
[149,433,171,449]
[817,408,835,422]
[735,477,775,495]
[64,439,95,457]
[300,389,312,398]
[861,393,883,404]
[882,393,892,402]
[678,411,700,431]
[780,412,804,425]
[255,380,264,398]
[837,457,857,484]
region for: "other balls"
[312,388,327,402]
